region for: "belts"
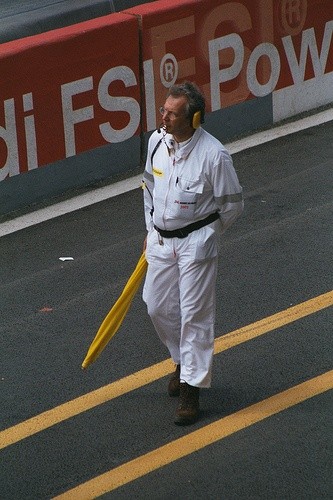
[155,213,220,238]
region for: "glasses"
[160,106,187,120]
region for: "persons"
[142,81,246,425]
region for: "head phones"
[179,87,205,129]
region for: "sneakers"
[174,384,199,425]
[168,363,181,398]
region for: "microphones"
[158,122,164,133]
